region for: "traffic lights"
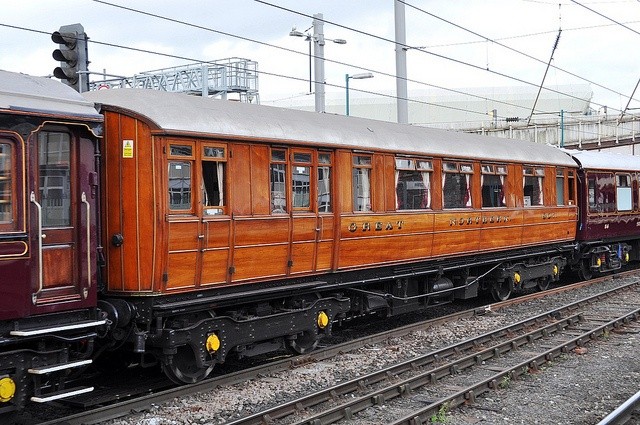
[52,24,87,93]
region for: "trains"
[0,71,637,424]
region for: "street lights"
[289,14,346,111]
[345,72,374,115]
